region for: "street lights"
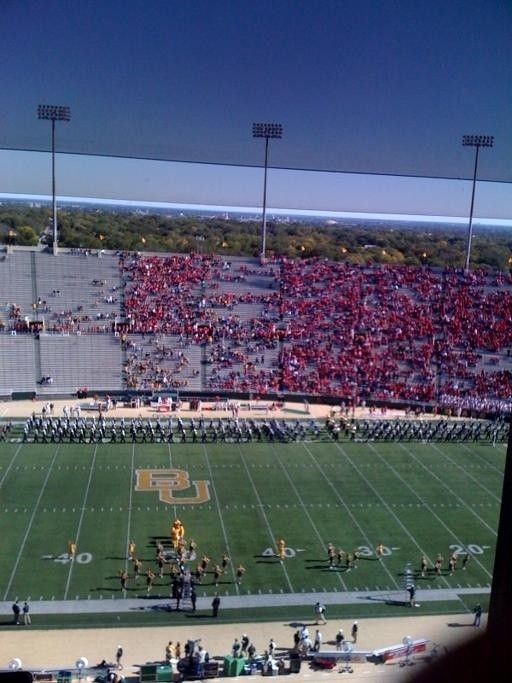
[252,121,283,255]
[37,103,71,242]
[461,133,495,270]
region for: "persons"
[474,602,481,627]
[116,519,246,618]
[279,536,286,561]
[117,621,359,679]
[420,552,470,576]
[67,539,76,561]
[376,543,386,558]
[12,601,32,625]
[315,603,326,625]
[328,541,358,567]
[1,246,512,444]
[407,585,417,608]
